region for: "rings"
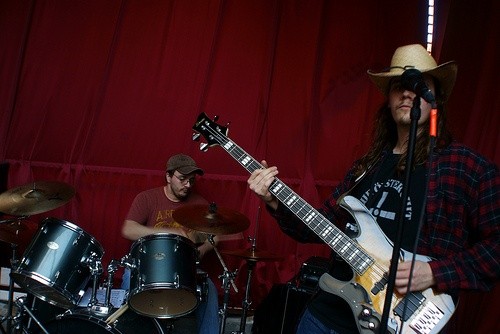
[263,182,268,189]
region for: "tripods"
[0,218,23,334]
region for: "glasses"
[172,173,196,184]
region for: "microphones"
[400,68,435,104]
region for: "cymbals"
[0,181,73,216]
[172,203,250,235]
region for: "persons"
[120,154,218,334]
[246,45,500,334]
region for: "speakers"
[279,285,313,334]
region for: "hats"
[165,153,204,176]
[366,44,458,106]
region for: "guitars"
[189,110,457,334]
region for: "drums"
[9,216,106,310]
[15,293,69,334]
[58,307,164,334]
[127,232,202,319]
